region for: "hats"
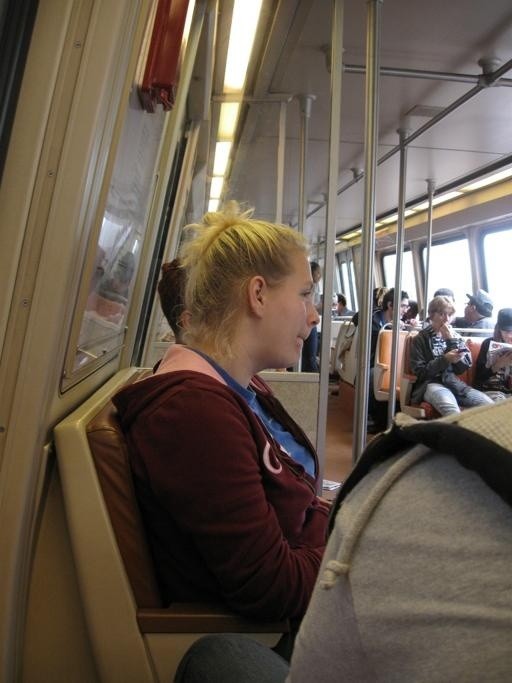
[498,309,511,331]
[466,289,493,311]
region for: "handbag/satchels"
[336,324,355,364]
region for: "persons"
[111,200,337,661]
[285,261,511,435]
[153,254,186,375]
[171,400,511,683]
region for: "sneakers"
[367,425,385,434]
[329,386,341,398]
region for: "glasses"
[400,305,411,310]
